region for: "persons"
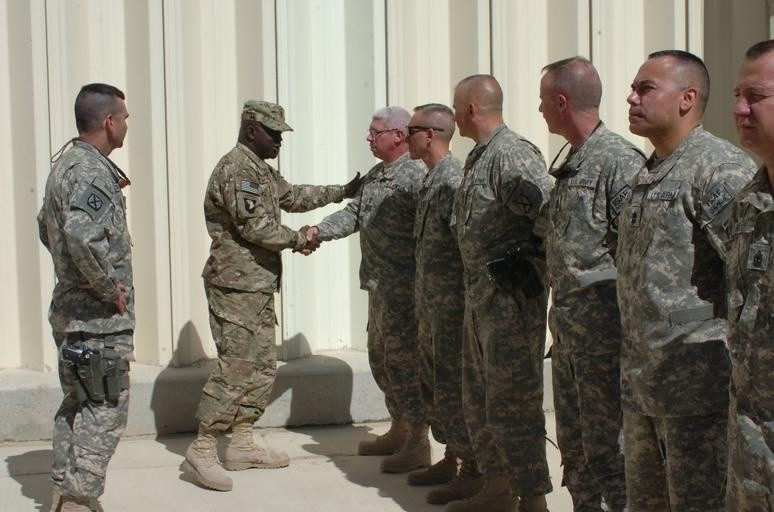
[38,83,135,512]
[725,39,774,512]
[184,100,366,492]
[619,50,756,512]
[302,105,431,473]
[406,102,483,505]
[443,74,554,511]
[531,59,645,511]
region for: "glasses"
[405,124,445,137]
[547,141,581,179]
[368,128,398,138]
[115,174,131,188]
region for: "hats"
[241,99,295,133]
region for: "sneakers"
[518,492,550,512]
[48,481,100,512]
[425,473,485,504]
[445,477,520,512]
[407,453,463,486]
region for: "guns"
[486,246,544,299]
[61,346,104,407]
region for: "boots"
[224,418,290,472]
[184,422,234,492]
[358,417,410,455]
[380,422,432,473]
